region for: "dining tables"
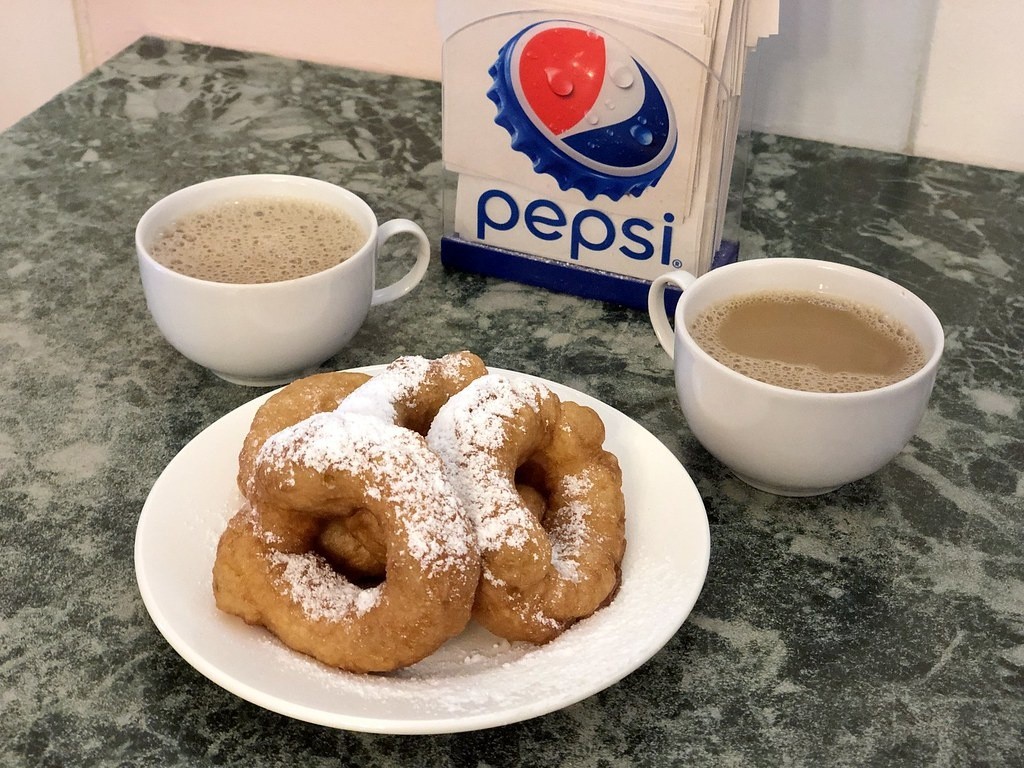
[1,34,1023,767]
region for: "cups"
[647,258,944,497]
[133,175,432,388]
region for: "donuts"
[210,350,629,676]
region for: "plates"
[132,363,712,737]
